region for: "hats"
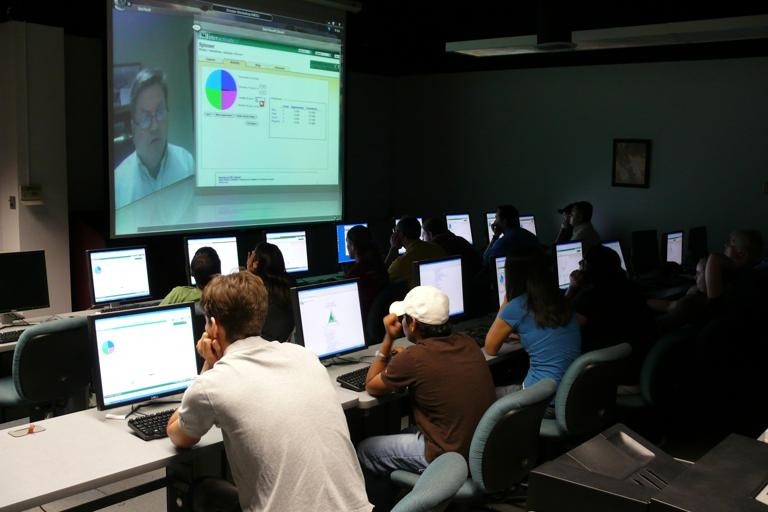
[558,203,574,212]
[389,286,450,325]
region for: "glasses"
[137,107,165,129]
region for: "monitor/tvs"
[85,244,153,310]
[262,226,312,287]
[519,215,536,236]
[662,230,683,265]
[687,226,708,264]
[552,240,583,289]
[602,241,628,271]
[334,222,369,274]
[289,277,368,361]
[87,300,201,419]
[0,250,50,324]
[494,256,509,307]
[445,213,473,246]
[183,232,243,287]
[485,212,504,248]
[631,229,661,270]
[392,217,423,255]
[412,254,466,321]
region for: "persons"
[245,243,294,310]
[645,228,768,440]
[337,199,601,346]
[484,243,584,420]
[114,68,196,211]
[572,240,658,386]
[360,284,495,475]
[157,247,223,305]
[166,270,376,511]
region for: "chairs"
[617,330,684,411]
[390,377,557,500]
[369,452,471,512]
[539,342,634,448]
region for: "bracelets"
[375,351,390,360]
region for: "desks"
[0,335,508,512]
[0,270,365,356]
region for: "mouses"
[471,334,485,348]
[508,333,520,340]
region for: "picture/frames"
[612,137,651,189]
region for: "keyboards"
[100,301,160,313]
[127,408,177,441]
[0,330,24,344]
[336,366,369,392]
